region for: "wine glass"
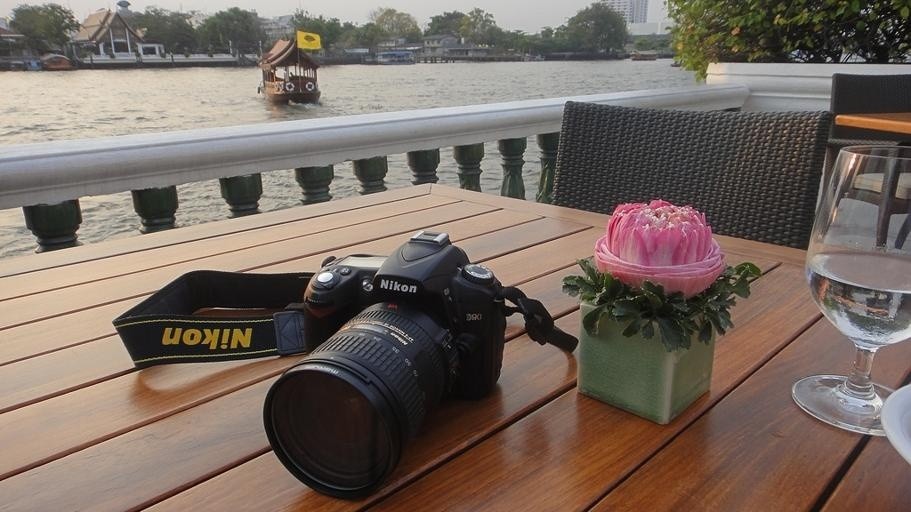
[790,147,911,439]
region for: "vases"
[572,299,717,426]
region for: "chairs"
[815,72,911,248]
[553,101,833,251]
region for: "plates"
[880,376,910,473]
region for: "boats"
[671,61,682,67]
[631,49,657,61]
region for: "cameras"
[262,230,508,501]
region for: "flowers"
[563,201,763,350]
